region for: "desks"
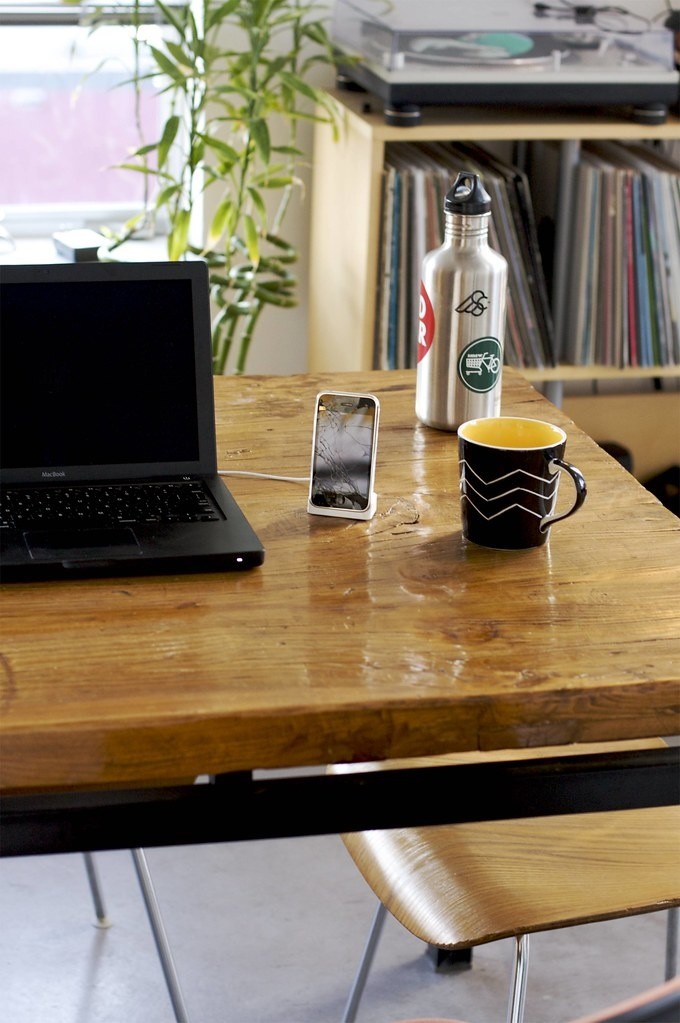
[0,364,680,859]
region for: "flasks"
[413,168,509,435]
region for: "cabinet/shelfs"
[308,85,680,481]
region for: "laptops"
[0,260,265,583]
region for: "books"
[374,141,679,370]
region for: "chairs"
[324,737,680,1023]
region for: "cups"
[456,417,588,550]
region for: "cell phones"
[308,391,380,513]
